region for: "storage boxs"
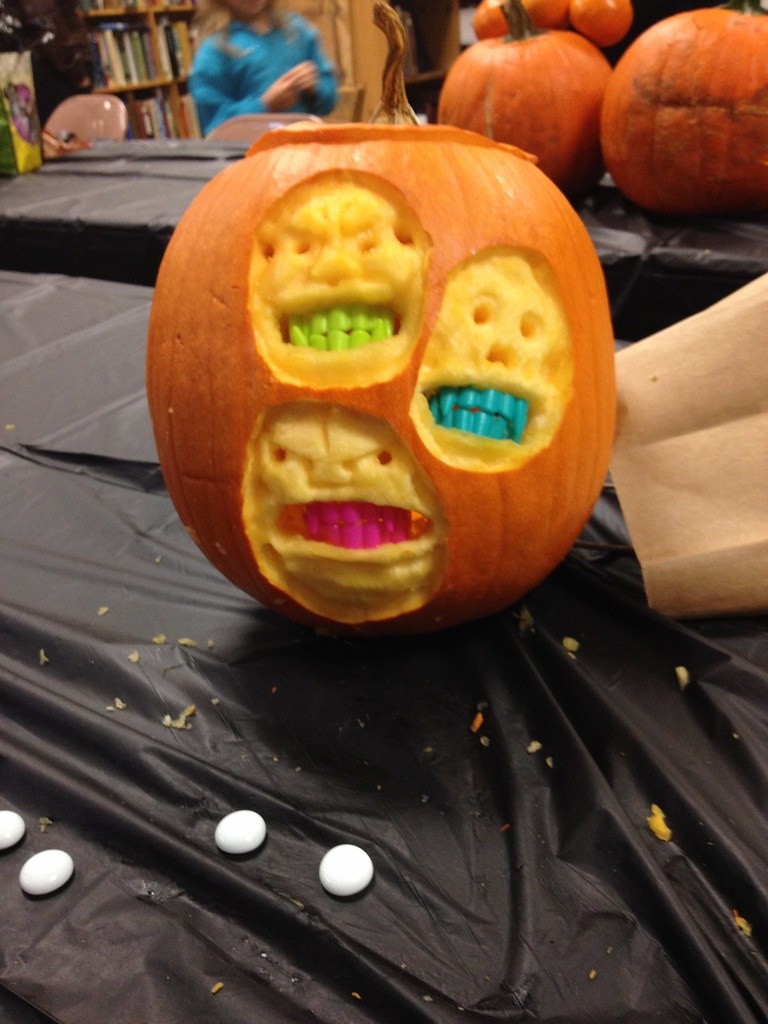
[0,50,42,176]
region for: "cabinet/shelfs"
[77,0,198,140]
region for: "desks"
[0,274,768,1024]
[0,138,765,336]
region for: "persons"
[187,0,337,136]
[0,0,98,128]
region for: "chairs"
[43,94,128,157]
[208,112,320,141]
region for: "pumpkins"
[434,0,768,217]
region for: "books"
[80,0,200,139]
[394,0,418,76]
